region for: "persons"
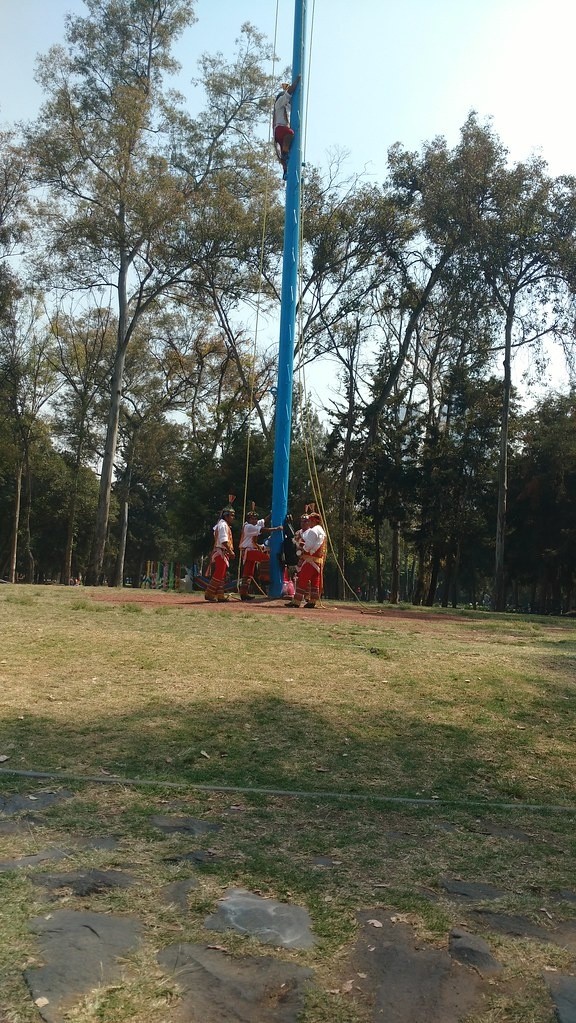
[285,503,328,608]
[239,502,283,600]
[204,494,236,602]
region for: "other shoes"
[205,595,217,601]
[285,602,300,608]
[218,598,229,602]
[304,602,315,608]
[241,595,256,601]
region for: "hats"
[308,503,321,522]
[301,505,310,522]
[222,495,235,516]
[246,502,259,520]
